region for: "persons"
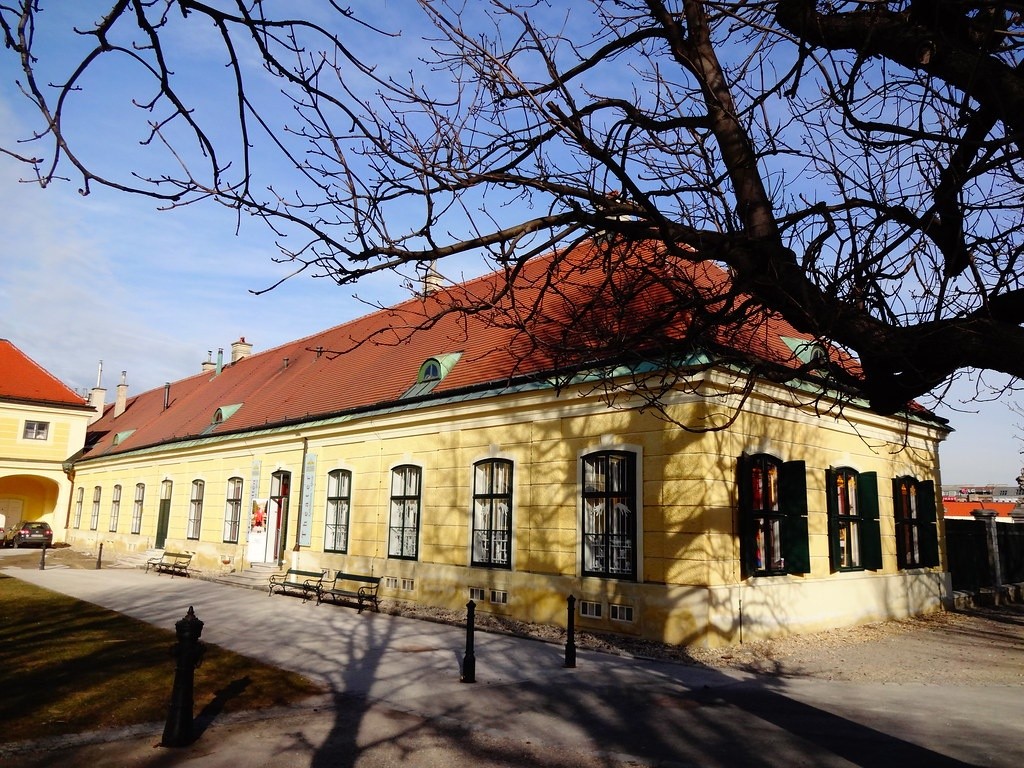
[254,505,263,533]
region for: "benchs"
[268,568,327,603]
[314,571,384,615]
[146,551,193,579]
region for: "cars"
[5,520,53,548]
